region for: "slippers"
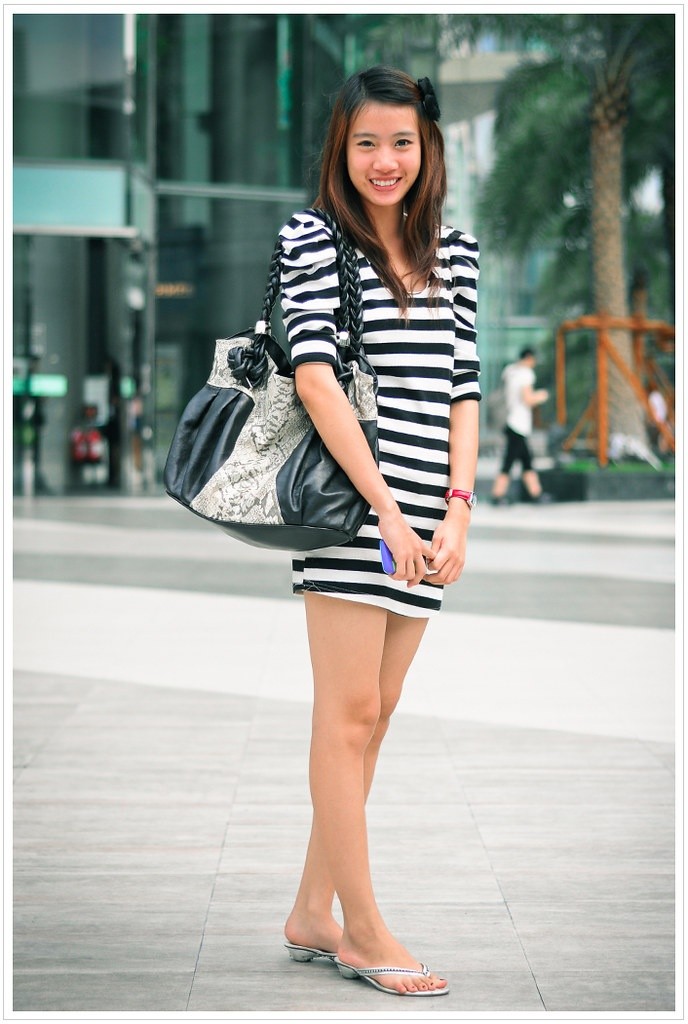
[287,943,335,961]
[333,956,449,997]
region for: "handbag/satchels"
[160,207,379,554]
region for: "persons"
[273,68,484,995]
[643,383,667,455]
[489,346,550,505]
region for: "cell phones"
[380,540,427,574]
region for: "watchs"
[444,488,478,510]
